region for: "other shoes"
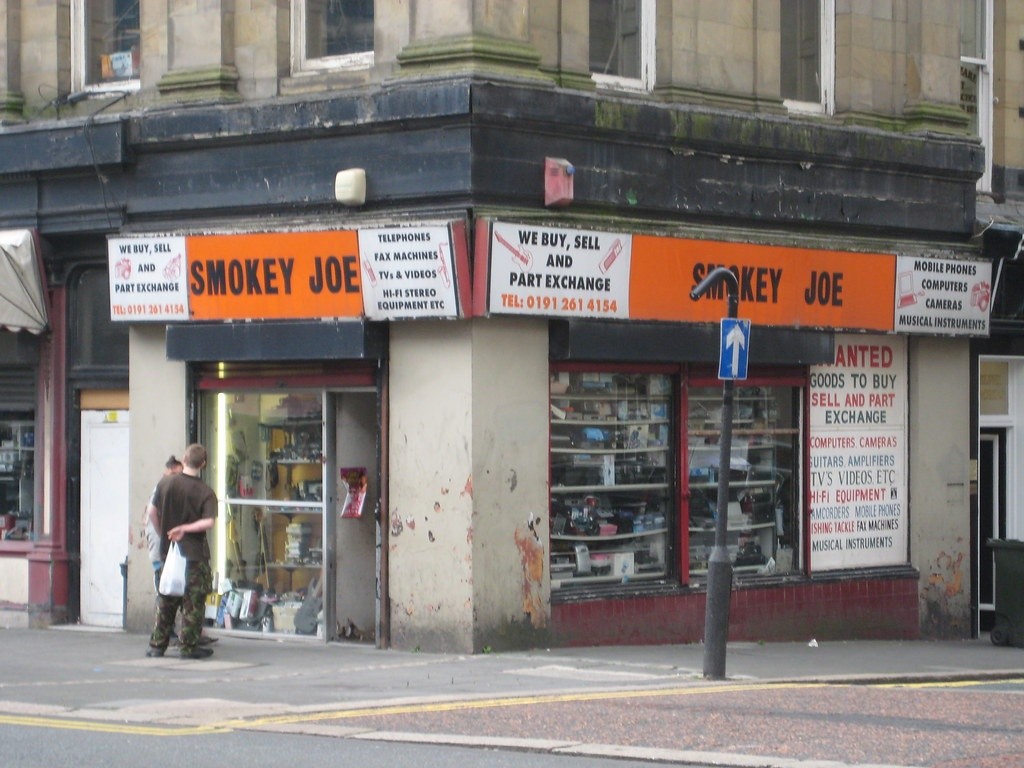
[145,645,165,658]
[181,647,214,660]
[198,635,219,646]
[167,633,180,647]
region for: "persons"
[146,441,215,660]
[144,456,220,645]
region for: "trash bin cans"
[989,535,1024,649]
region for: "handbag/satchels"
[158,541,187,598]
